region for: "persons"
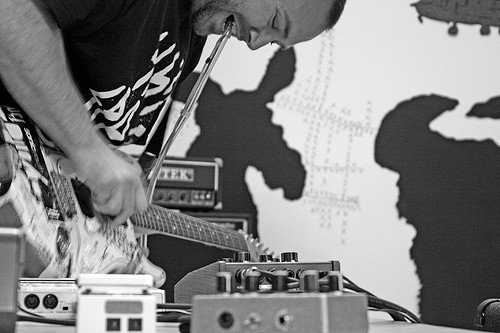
[1,0,346,229]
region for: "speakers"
[146,213,255,303]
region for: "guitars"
[0,118,274,287]
